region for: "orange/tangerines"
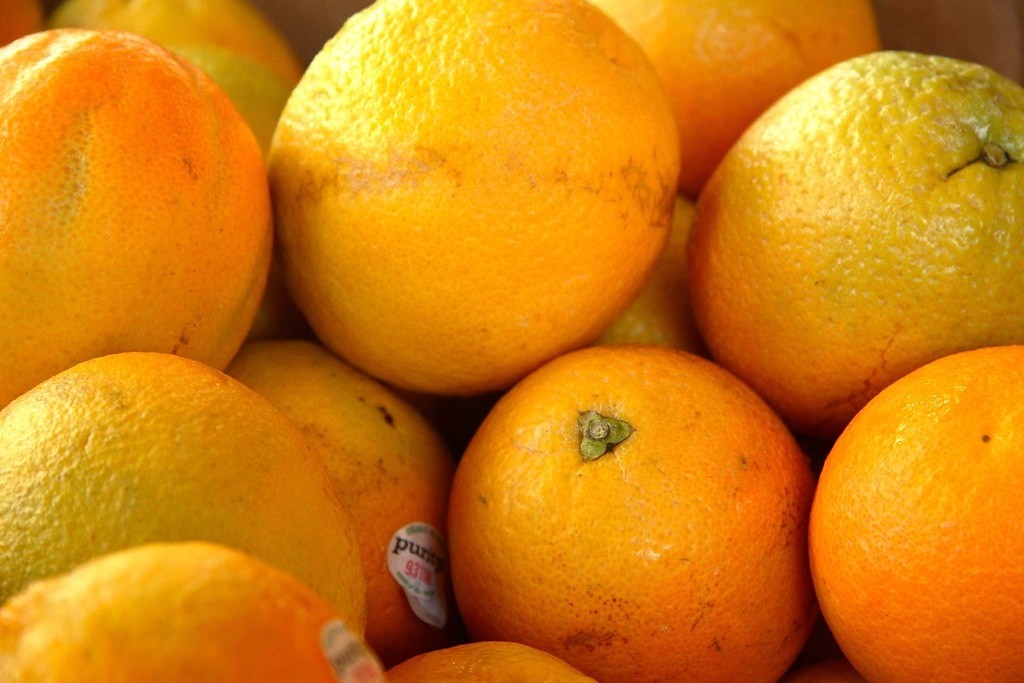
[0,0,1024,683]
